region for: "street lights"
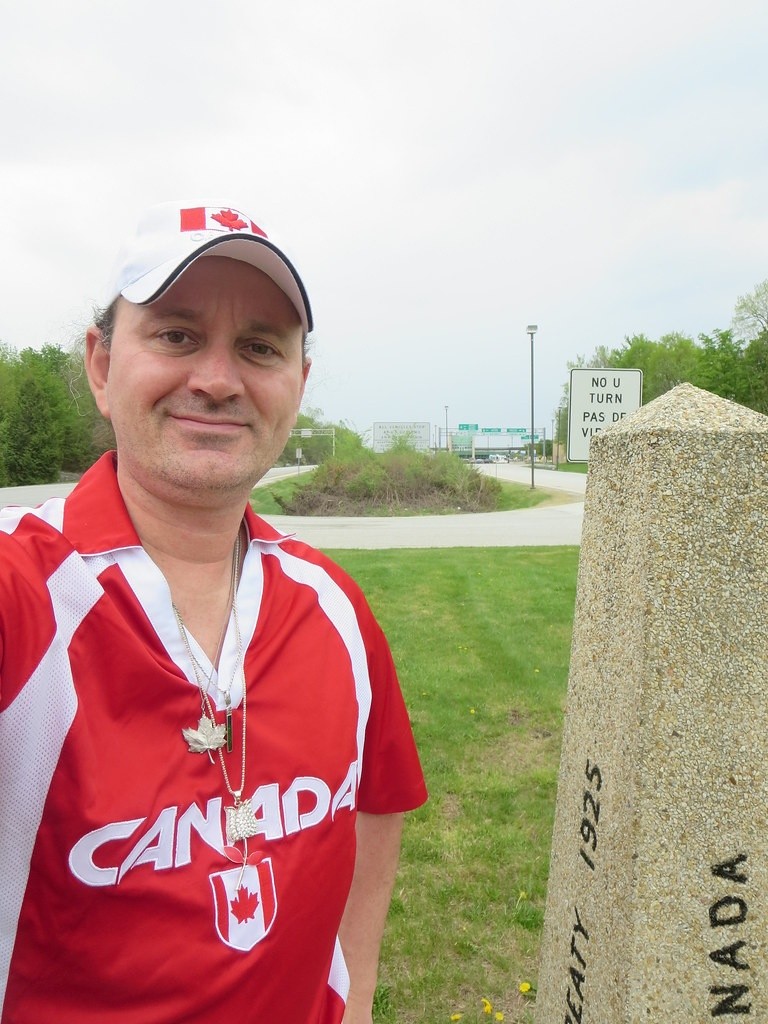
[551,417,554,464]
[526,324,539,491]
[555,406,562,469]
[445,405,448,452]
[434,425,437,449]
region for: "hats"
[94,204,315,339]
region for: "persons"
[0,199,429,1024]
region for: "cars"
[462,455,508,464]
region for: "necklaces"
[171,518,263,893]
